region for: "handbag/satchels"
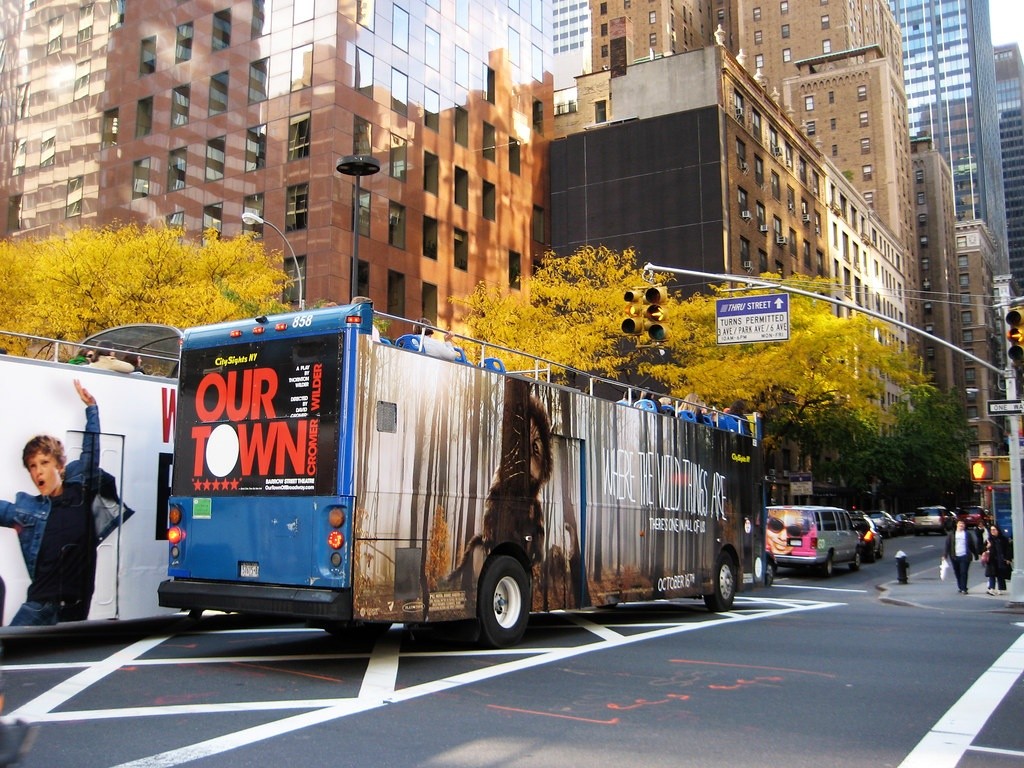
[981,550,990,567]
[940,559,949,581]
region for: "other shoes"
[998,589,1006,594]
[961,590,966,594]
[987,589,994,595]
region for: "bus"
[0,324,191,635]
[156,303,767,653]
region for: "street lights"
[335,155,381,299]
[241,213,305,311]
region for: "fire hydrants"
[894,550,912,585]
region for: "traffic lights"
[621,291,644,337]
[1007,306,1024,367]
[970,459,994,482]
[646,287,669,341]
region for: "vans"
[764,504,865,580]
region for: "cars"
[912,506,956,535]
[850,508,915,566]
[956,504,992,529]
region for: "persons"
[658,396,672,406]
[122,351,146,374]
[727,399,753,436]
[411,317,456,361]
[0,378,136,627]
[67,347,99,365]
[320,301,338,308]
[671,391,731,431]
[351,296,381,343]
[765,509,811,555]
[942,518,989,594]
[984,524,1013,596]
[670,393,704,424]
[89,340,135,372]
[616,388,640,408]
[630,388,665,414]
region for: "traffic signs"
[986,400,1024,416]
[715,292,790,344]
